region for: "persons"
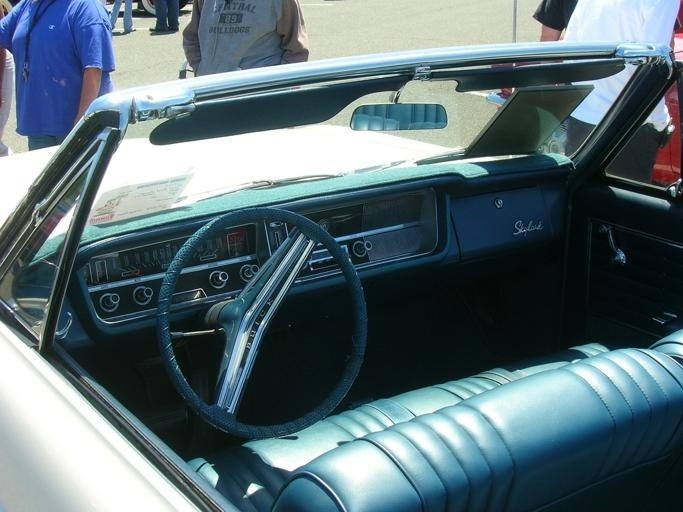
[0,0,14,157]
[111,0,136,33]
[0,0,115,151]
[532,0,681,185]
[149,0,179,34]
[182,0,309,78]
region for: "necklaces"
[21,0,55,81]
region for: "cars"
[0,37,683,511]
[482,0,683,190]
[138,0,192,18]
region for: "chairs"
[180,347,682,511]
[469,328,682,403]
